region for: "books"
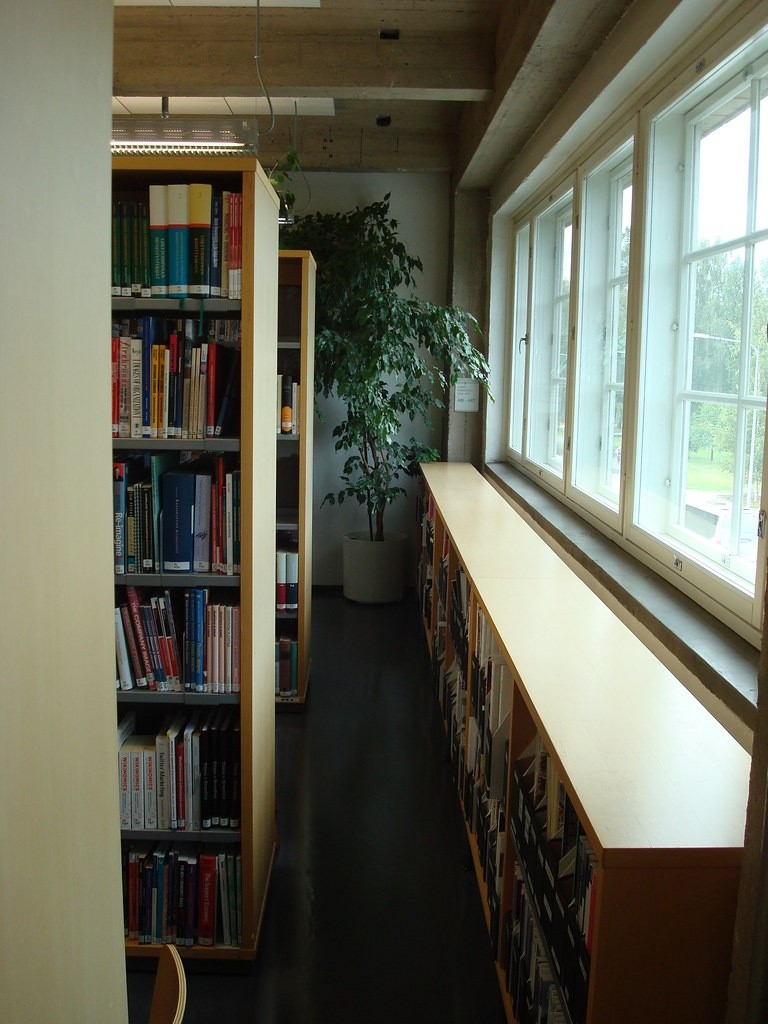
[502,733,600,1024]
[275,543,299,613]
[112,315,240,438]
[274,634,298,698]
[462,602,514,968]
[431,529,471,802]
[111,181,241,301]
[278,373,299,436]
[114,588,241,695]
[420,492,434,634]
[112,452,241,576]
[121,843,242,946]
[117,709,241,829]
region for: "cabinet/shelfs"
[0,0,316,1024]
[418,460,752,1024]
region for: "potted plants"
[276,191,495,605]
